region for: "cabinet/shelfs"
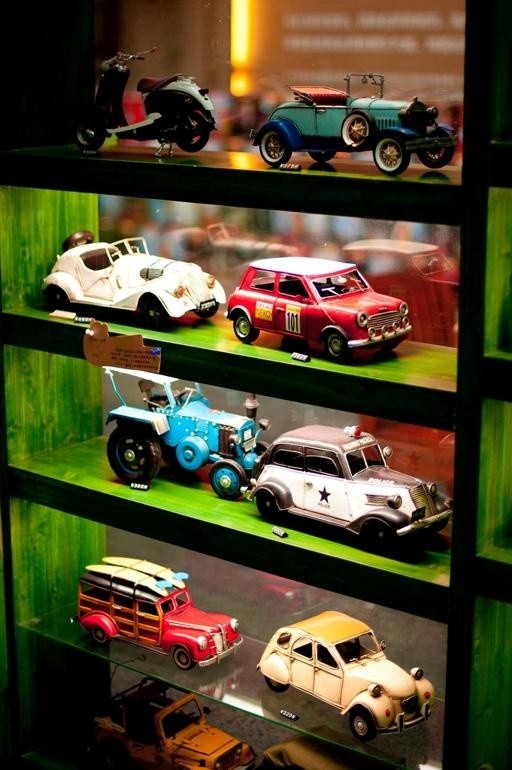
[1,3,509,769]
